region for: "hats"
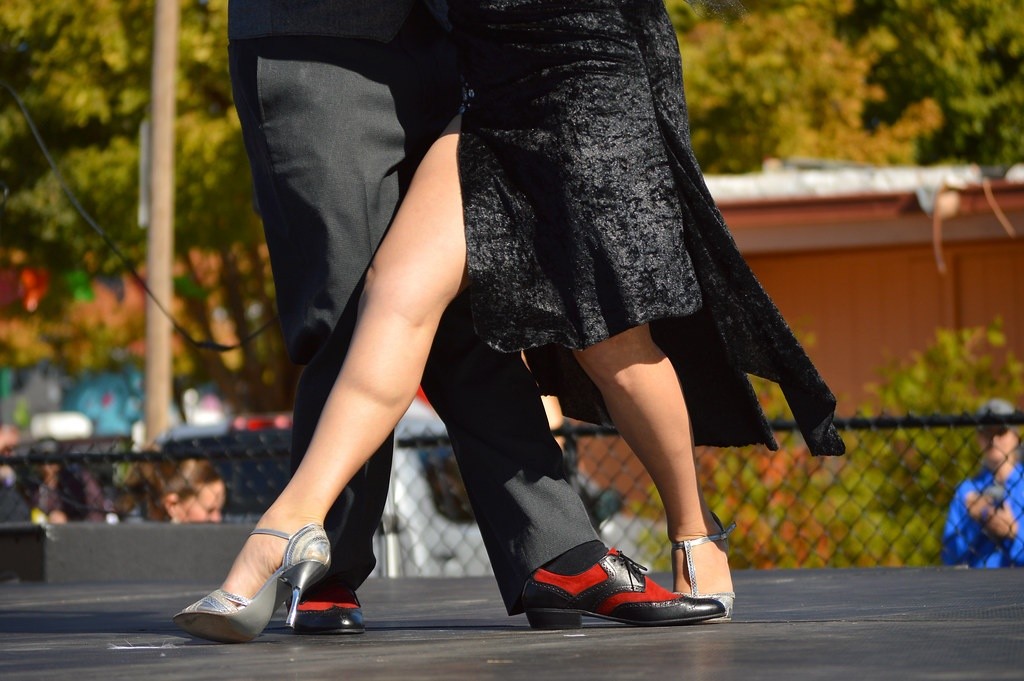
[978,400,1016,429]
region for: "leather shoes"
[522,548,728,630]
[286,585,365,635]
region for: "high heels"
[672,511,737,623]
[173,522,331,643]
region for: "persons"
[943,398,1024,568]
[0,454,225,524]
[172,1,736,639]
